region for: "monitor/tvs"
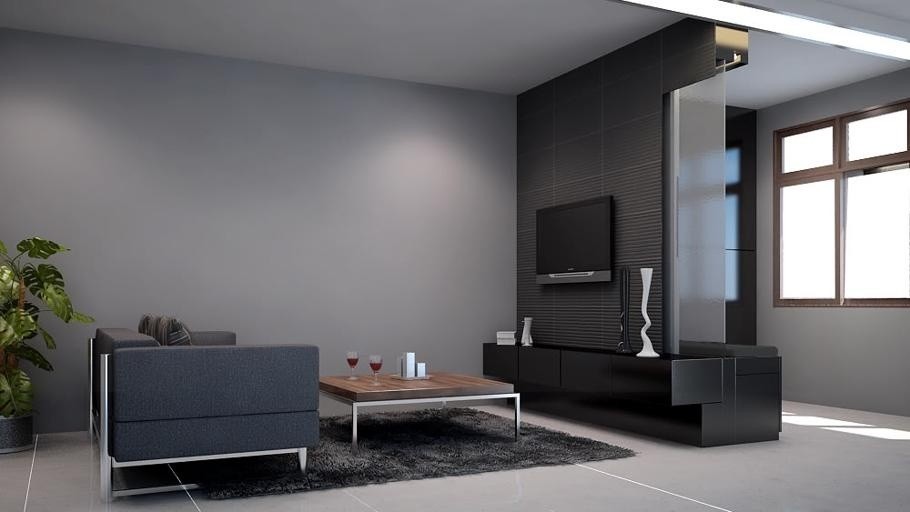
[536,195,616,284]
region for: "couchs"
[87,328,320,500]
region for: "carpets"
[185,405,641,502]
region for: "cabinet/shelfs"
[482,342,782,448]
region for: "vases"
[617,267,660,356]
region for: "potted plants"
[0,235,96,454]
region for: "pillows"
[138,313,193,346]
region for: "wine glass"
[344,351,360,381]
[368,354,383,387]
